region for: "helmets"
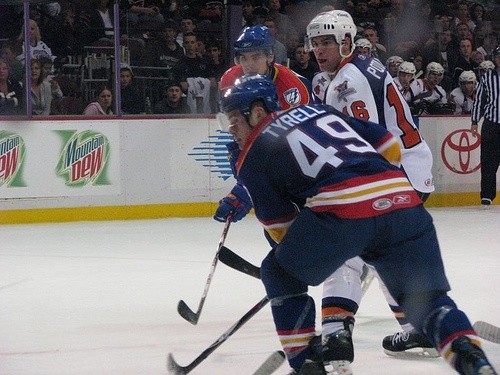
[459,70,476,85]
[399,61,416,73]
[222,74,282,115]
[479,60,495,70]
[426,62,444,73]
[493,47,500,55]
[234,25,274,51]
[307,10,357,58]
[386,56,404,69]
[355,39,372,54]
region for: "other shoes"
[481,197,493,205]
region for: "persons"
[0,0,228,115]
[219,8,443,375]
[242,0,321,81]
[471,45,500,208]
[214,72,498,375]
[320,0,500,115]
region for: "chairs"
[50,78,85,113]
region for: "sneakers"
[287,335,327,375]
[319,316,355,375]
[382,328,440,360]
[451,337,497,375]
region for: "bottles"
[144,97,153,114]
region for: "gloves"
[214,183,253,223]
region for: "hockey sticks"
[217,245,263,280]
[402,69,424,97]
[177,211,233,325]
[253,350,286,375]
[167,295,270,375]
[410,92,434,103]
[472,321,500,344]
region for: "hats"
[120,63,133,74]
[164,80,181,91]
